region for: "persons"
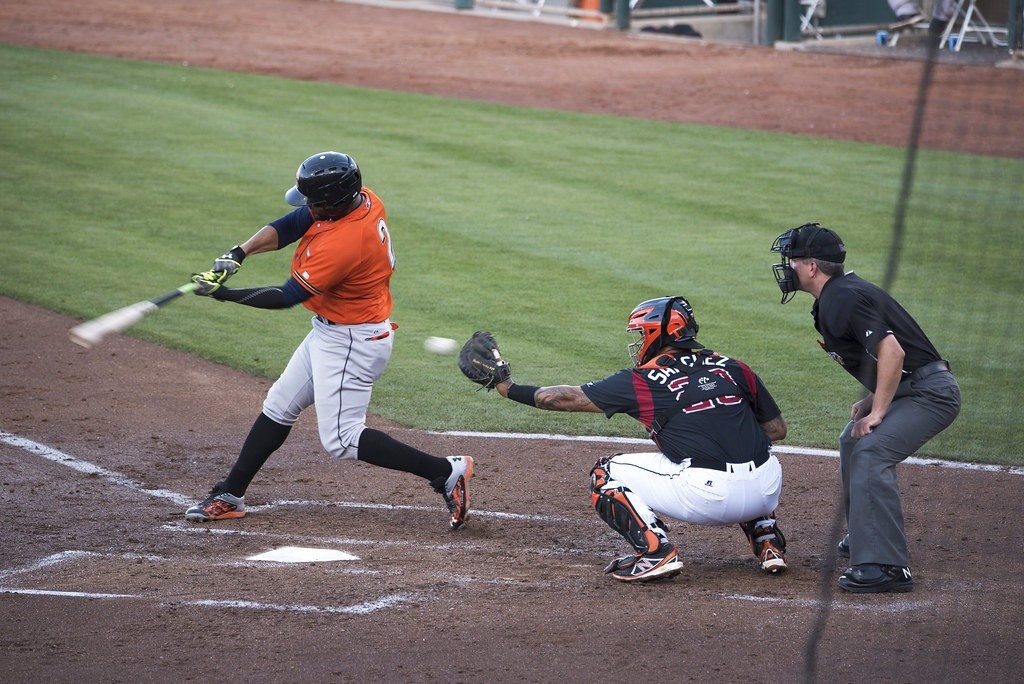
[770,222,962,594]
[184,151,475,532]
[457,293,788,581]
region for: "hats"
[785,225,846,263]
[669,336,705,349]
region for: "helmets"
[285,151,362,209]
[626,296,693,367]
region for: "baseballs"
[423,337,458,356]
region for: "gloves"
[211,246,245,284]
[191,271,227,302]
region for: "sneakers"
[837,562,914,593]
[185,482,245,521]
[839,533,851,557]
[749,529,788,573]
[612,543,683,580]
[429,455,473,531]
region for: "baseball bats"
[67,284,204,351]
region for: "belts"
[910,361,948,381]
[316,313,335,325]
[690,448,770,473]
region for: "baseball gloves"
[457,329,512,390]
